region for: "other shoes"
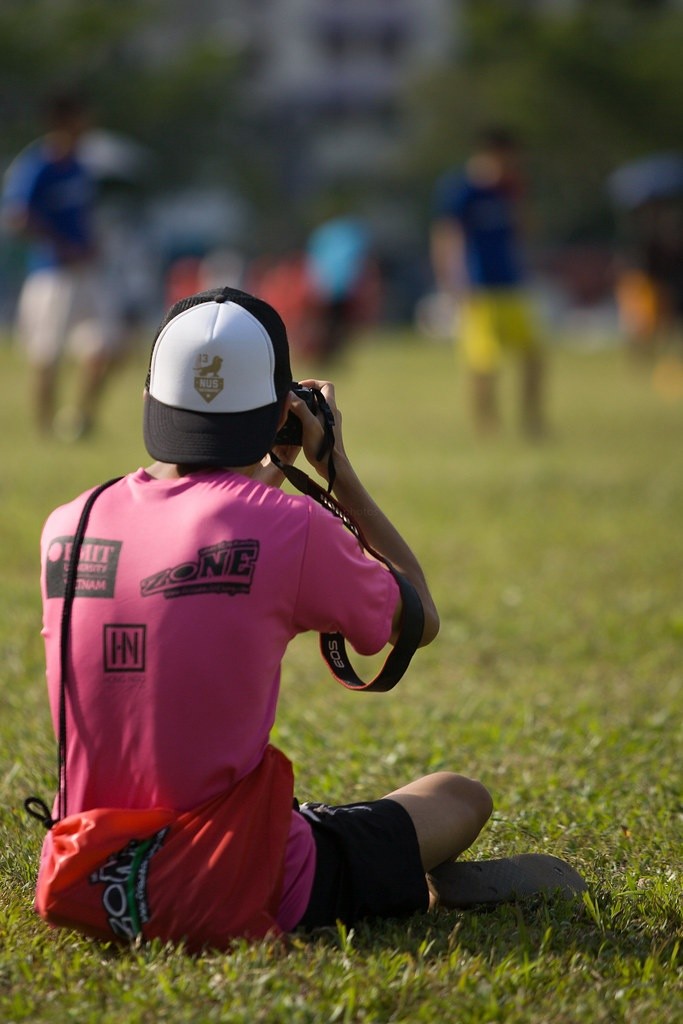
[425,854,588,912]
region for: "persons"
[0,97,681,445]
[41,285,591,935]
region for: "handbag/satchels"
[24,744,296,954]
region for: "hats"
[142,287,292,468]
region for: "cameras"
[270,381,316,445]
